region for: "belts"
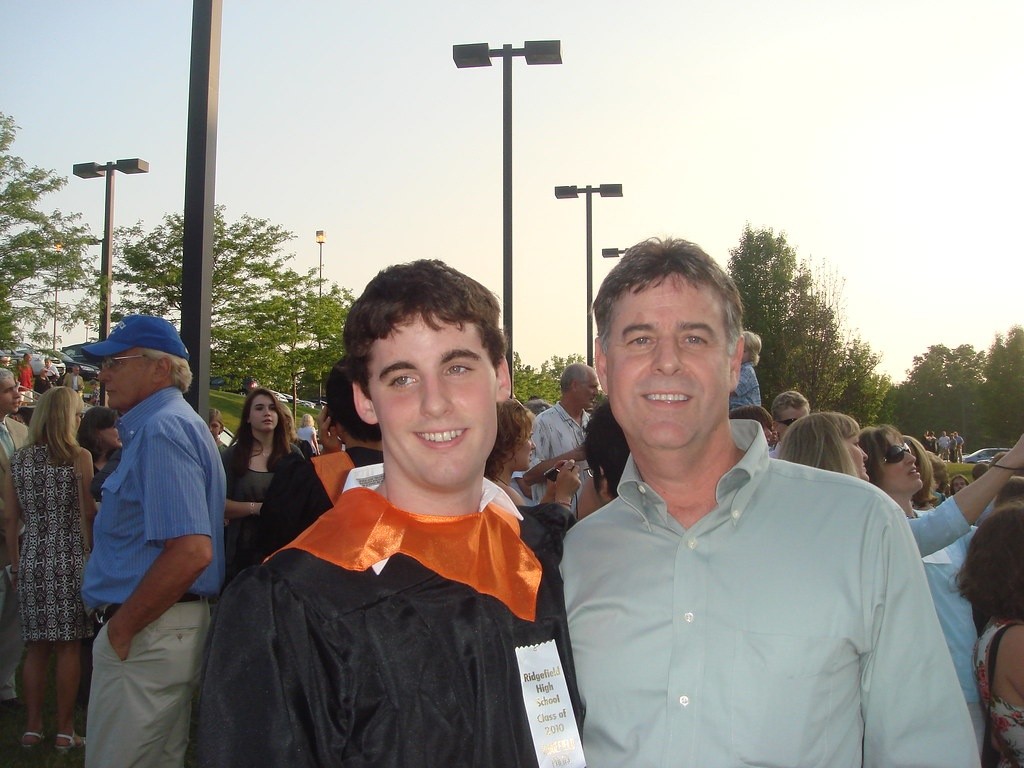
[95,592,204,625]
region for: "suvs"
[0,340,66,382]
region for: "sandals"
[54,730,86,753]
[22,730,44,749]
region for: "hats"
[81,316,189,365]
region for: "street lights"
[555,184,623,362]
[453,40,562,401]
[316,230,324,405]
[53,242,63,350]
[73,158,150,406]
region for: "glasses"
[104,354,146,370]
[885,442,912,464]
[778,418,796,427]
[319,399,329,409]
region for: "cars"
[38,349,100,380]
[308,400,327,405]
[61,344,99,367]
[268,390,315,409]
[210,373,260,395]
[977,457,994,464]
[963,447,1013,464]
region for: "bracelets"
[250,503,255,514]
[10,566,18,574]
[556,501,572,508]
[994,464,1019,471]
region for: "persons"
[729,331,762,410]
[87,381,100,406]
[561,236,982,768]
[5,386,97,751]
[63,366,83,397]
[956,503,1024,768]
[209,409,228,454]
[80,315,225,768]
[275,355,627,558]
[222,389,305,582]
[16,353,60,402]
[731,391,1024,752]
[0,369,29,704]
[90,449,122,502]
[78,406,122,477]
[190,258,585,768]
